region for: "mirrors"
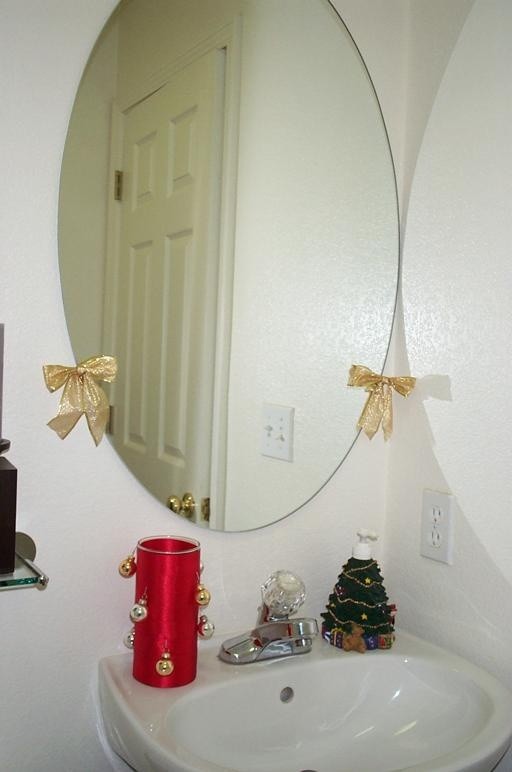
[55,0,403,540]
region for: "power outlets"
[260,399,294,465]
[417,489,455,567]
